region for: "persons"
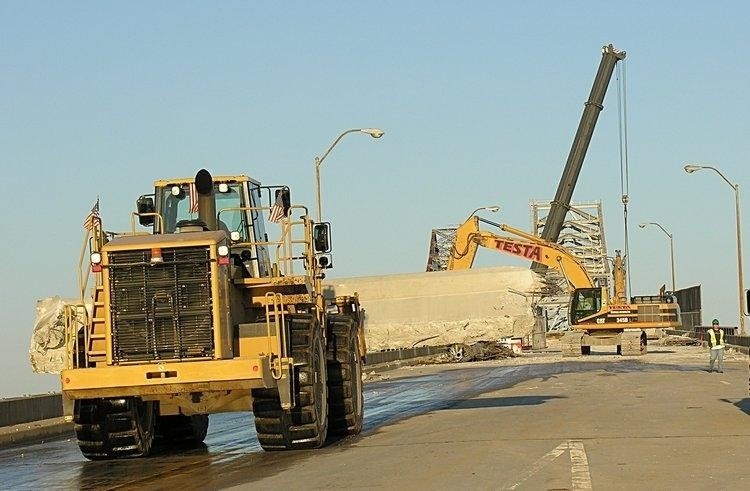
[706,319,724,374]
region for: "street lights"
[314,129,388,281]
[683,164,746,336]
[461,205,504,221]
[638,222,676,294]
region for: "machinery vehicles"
[29,169,368,460]
[448,218,683,358]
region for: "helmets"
[712,318,719,323]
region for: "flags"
[267,194,285,225]
[188,183,199,214]
[82,200,99,231]
